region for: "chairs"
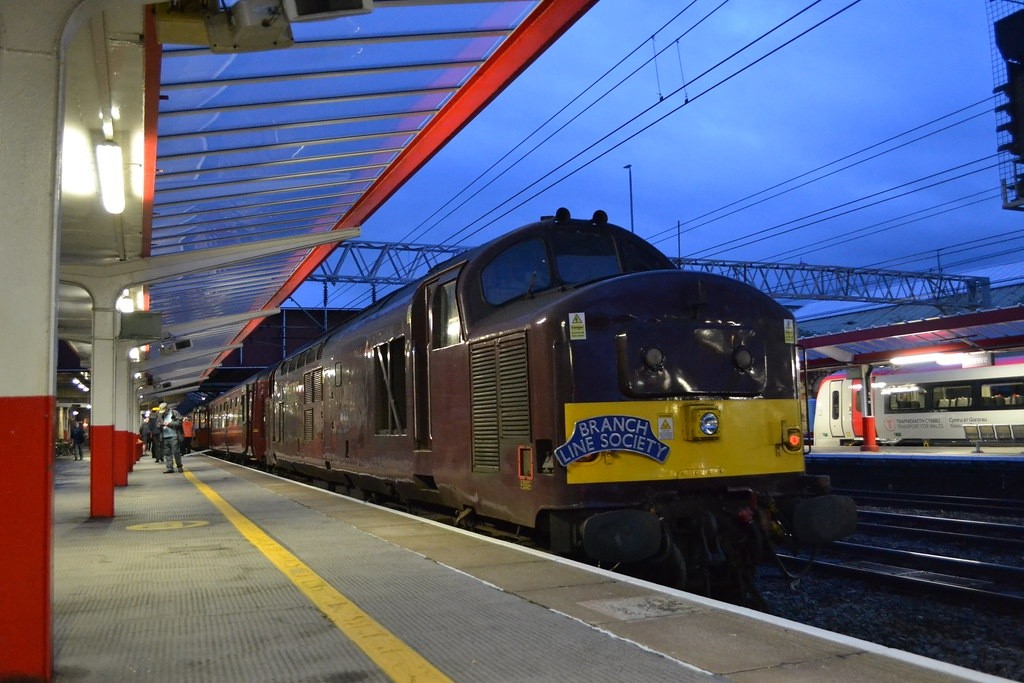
[891,394,1024,410]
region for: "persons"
[156,402,184,474]
[71,422,86,461]
[139,407,193,462]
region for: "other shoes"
[163,470,174,473]
[161,459,164,461]
[155,460,159,463]
[178,467,183,472]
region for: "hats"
[158,402,168,413]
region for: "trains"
[813,348,1024,448]
[187,206,815,587]
